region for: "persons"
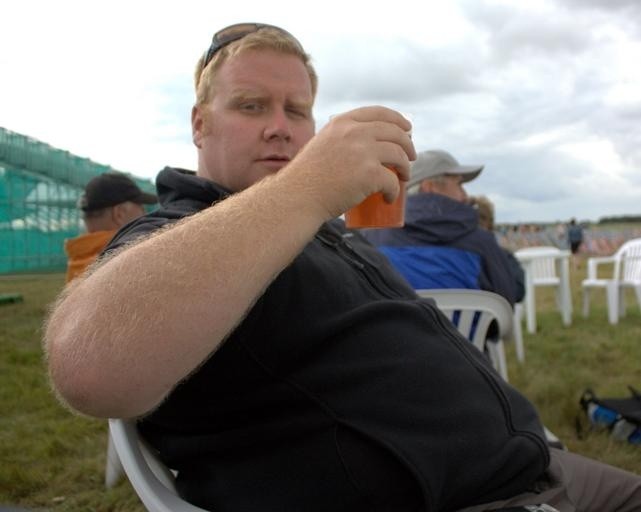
[567,218,582,271]
[351,148,517,374]
[579,220,594,257]
[59,167,160,285]
[39,19,639,511]
[468,196,528,344]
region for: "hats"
[79,172,158,211]
[405,148,484,191]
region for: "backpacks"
[575,381,641,447]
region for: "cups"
[344,127,411,232]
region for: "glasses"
[202,21,305,72]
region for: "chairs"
[106,418,210,512]
[413,288,514,384]
[513,246,573,334]
[582,238,641,324]
